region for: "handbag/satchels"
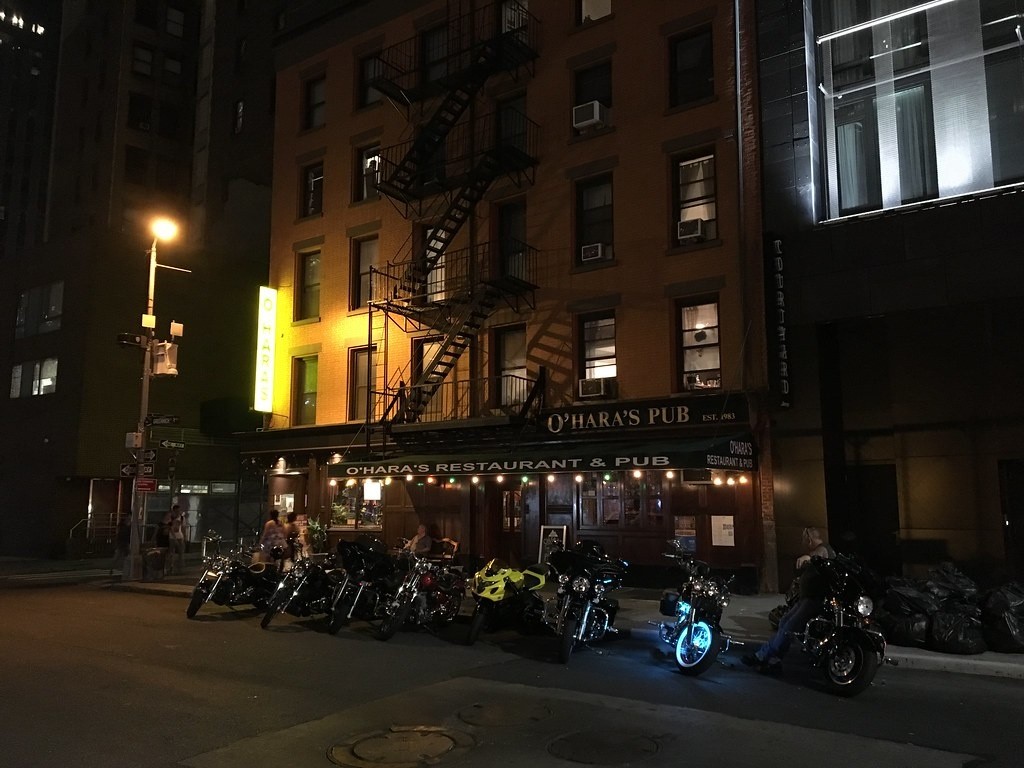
[252,547,266,564]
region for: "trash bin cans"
[142,547,168,583]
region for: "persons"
[162,504,187,571]
[260,510,300,571]
[403,524,432,554]
[110,512,132,576]
[741,527,836,666]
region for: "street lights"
[129,216,183,581]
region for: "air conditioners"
[573,101,608,131]
[579,377,610,399]
[678,218,706,240]
[136,122,150,130]
[582,243,606,262]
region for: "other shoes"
[741,654,761,667]
[753,647,761,652]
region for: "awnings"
[327,431,760,473]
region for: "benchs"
[398,538,459,562]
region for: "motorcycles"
[647,538,746,676]
[768,551,887,699]
[542,558,628,666]
[186,527,285,618]
[467,558,552,646]
[260,538,469,642]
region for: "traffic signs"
[159,439,185,450]
[120,462,155,478]
[129,448,158,464]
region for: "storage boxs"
[578,540,604,557]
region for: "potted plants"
[307,514,328,564]
